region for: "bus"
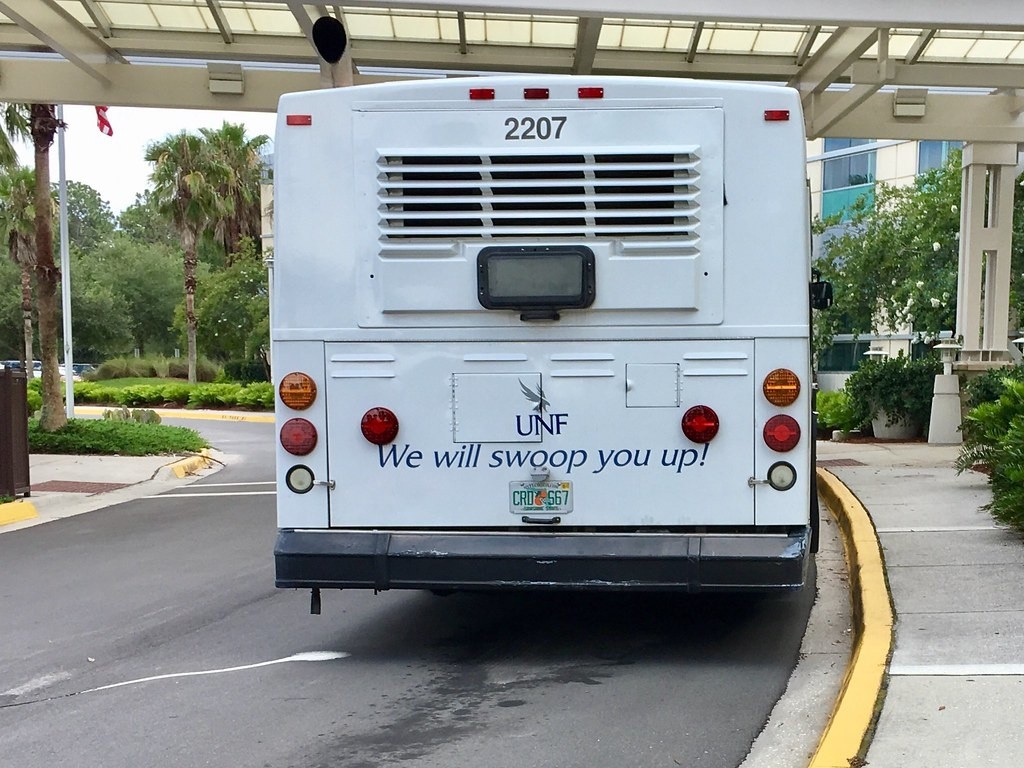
[270,74,832,596]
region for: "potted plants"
[844,348,944,440]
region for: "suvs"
[0,362,26,380]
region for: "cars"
[73,364,95,376]
[25,361,80,383]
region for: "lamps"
[893,88,928,116]
[863,346,890,363]
[934,338,962,375]
[206,62,245,93]
